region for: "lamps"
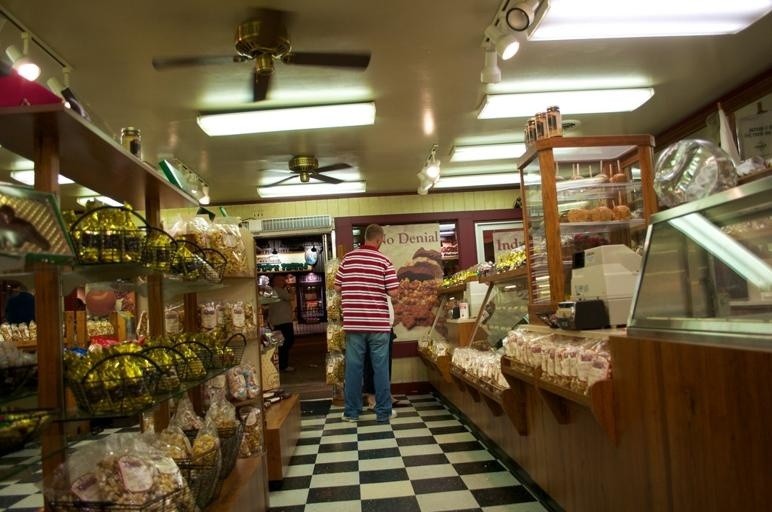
[480,0,540,85]
[4,32,72,104]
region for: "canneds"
[524,105,562,150]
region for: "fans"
[150,9,372,102]
[258,155,352,190]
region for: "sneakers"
[341,412,359,422]
[280,367,295,372]
[391,409,397,418]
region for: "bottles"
[121,126,141,163]
[524,105,564,150]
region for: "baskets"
[139,344,190,394]
[211,332,247,371]
[170,240,207,280]
[69,352,166,415]
[0,361,61,455]
[136,226,179,273]
[183,431,237,499]
[184,420,244,478]
[192,249,227,283]
[166,339,213,381]
[69,206,149,264]
[47,463,217,512]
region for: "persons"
[285,273,295,284]
[333,224,402,423]
[267,275,298,374]
[362,290,400,411]
